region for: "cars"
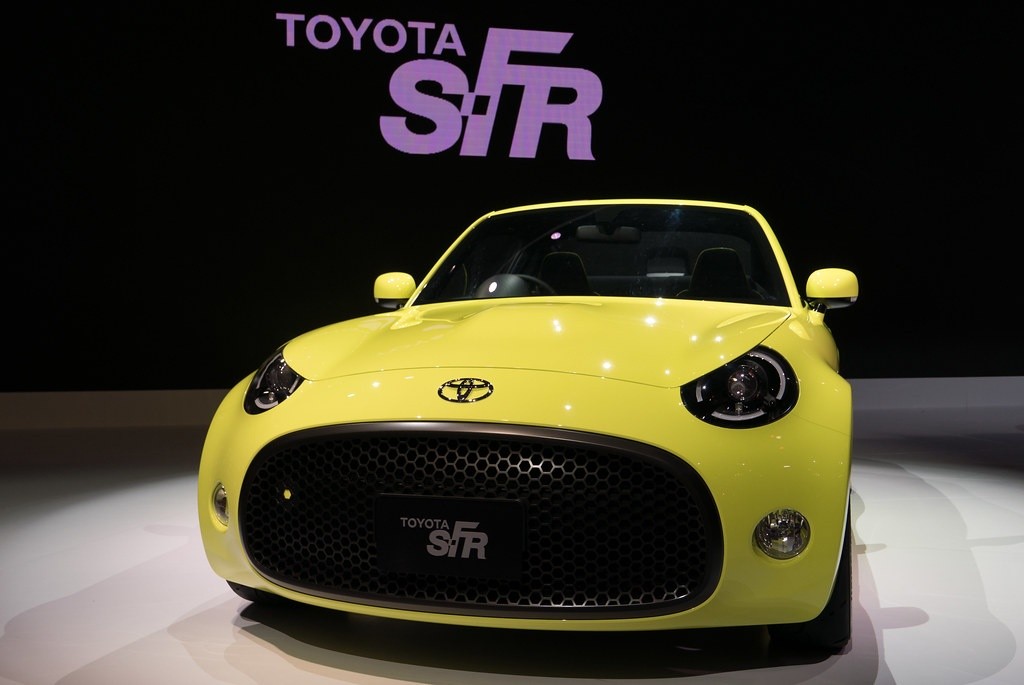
[195,198,861,658]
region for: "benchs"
[586,274,777,304]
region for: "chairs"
[531,251,600,296]
[675,246,766,305]
